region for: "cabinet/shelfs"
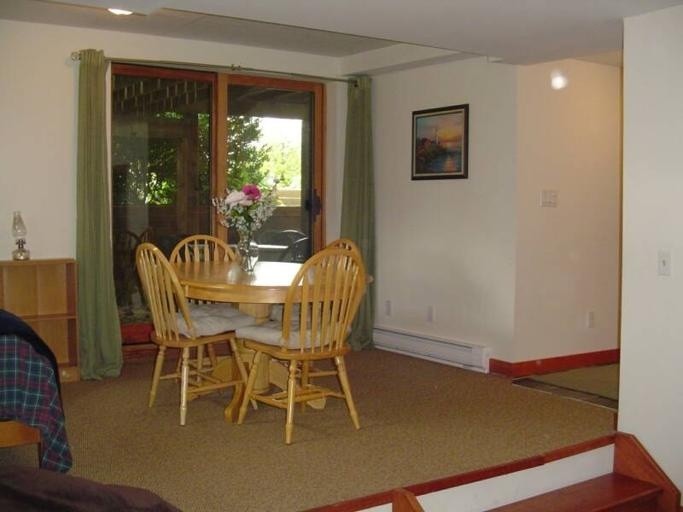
[0,259,79,384]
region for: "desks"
[153,258,374,424]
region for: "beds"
[0,300,78,471]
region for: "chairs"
[233,247,368,447]
[132,237,261,425]
[164,232,245,396]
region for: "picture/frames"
[411,102,470,180]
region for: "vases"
[231,229,253,274]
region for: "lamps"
[10,207,28,261]
[541,62,570,92]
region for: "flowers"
[211,181,282,264]
